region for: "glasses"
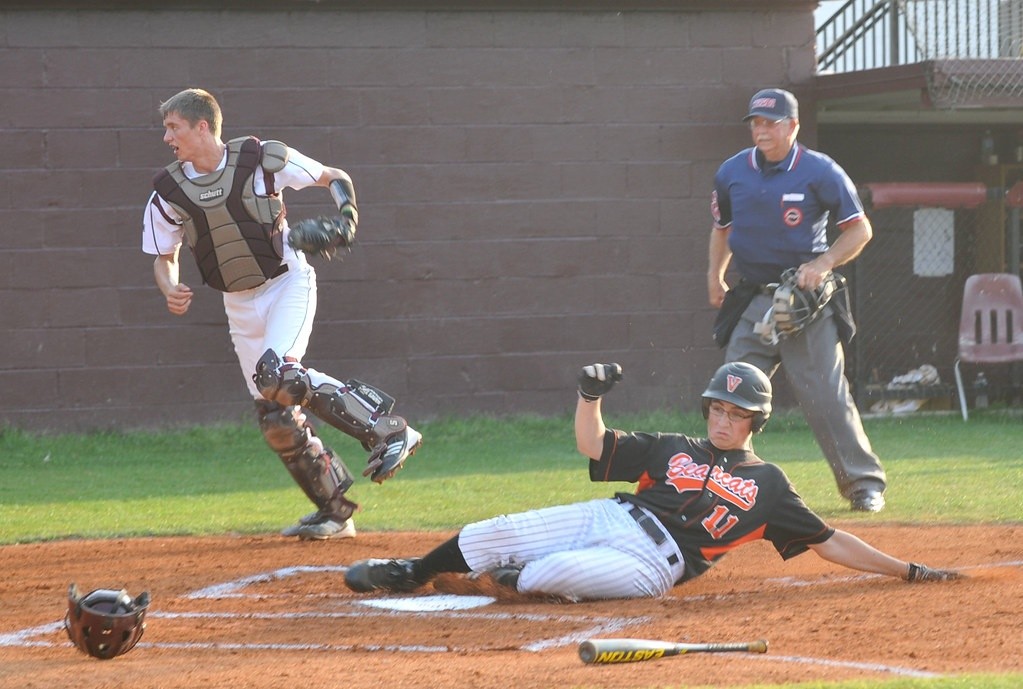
[751,114,791,129]
[708,402,757,422]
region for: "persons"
[142,89,422,540]
[342,361,961,600]
[707,88,889,514]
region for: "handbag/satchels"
[712,278,760,351]
[828,287,856,344]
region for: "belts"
[227,263,288,294]
[612,495,679,566]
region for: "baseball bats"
[578,639,768,664]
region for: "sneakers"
[490,565,520,593]
[342,557,426,595]
[297,516,356,540]
[371,425,423,484]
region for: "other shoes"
[851,489,886,514]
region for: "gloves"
[575,362,622,402]
[328,179,359,247]
[916,566,968,582]
[285,215,346,259]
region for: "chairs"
[953,272,1023,421]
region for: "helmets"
[64,582,148,660]
[753,268,846,346]
[702,361,773,434]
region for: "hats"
[743,88,800,122]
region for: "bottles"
[975,371,988,409]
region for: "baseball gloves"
[773,268,846,337]
[289,218,356,262]
[65,581,150,659]
